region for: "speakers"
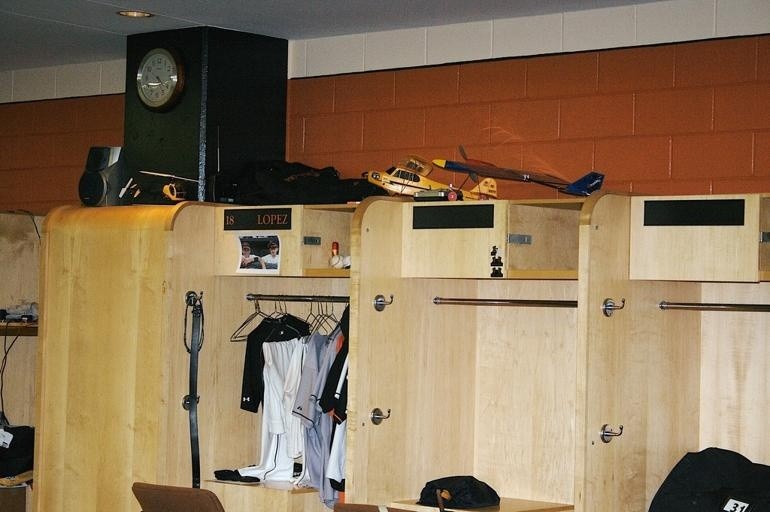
[77,146,120,207]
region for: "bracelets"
[254,257,258,262]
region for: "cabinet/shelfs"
[0,193,769,512]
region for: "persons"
[241,242,260,267]
[260,242,279,269]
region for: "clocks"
[134,47,187,113]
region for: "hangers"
[230,294,338,345]
